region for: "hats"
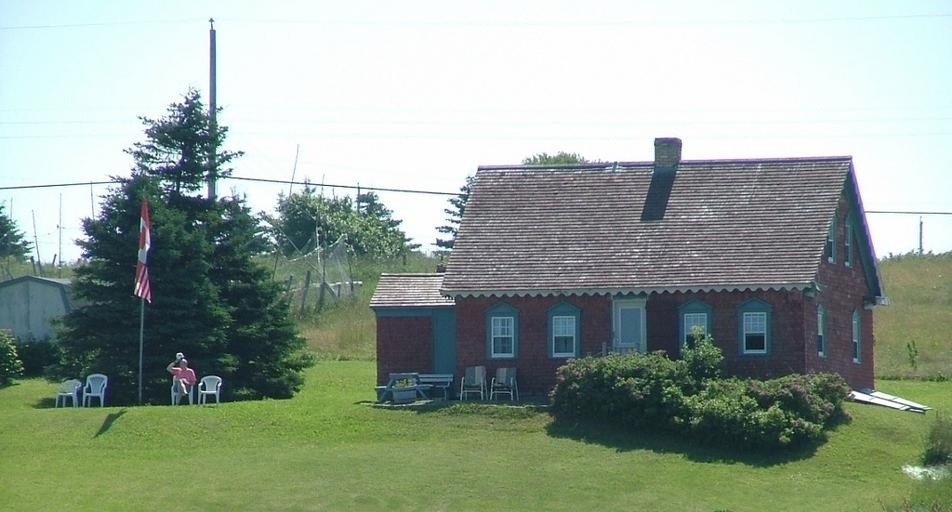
[176,352,185,360]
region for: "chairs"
[460,365,488,401]
[56,378,82,407]
[490,367,519,402]
[198,375,222,406]
[171,375,193,406]
[82,374,108,407]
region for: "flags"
[138,197,151,263]
[134,259,152,304]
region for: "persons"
[166,351,196,405]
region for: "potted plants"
[392,376,418,403]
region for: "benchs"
[375,373,454,403]
